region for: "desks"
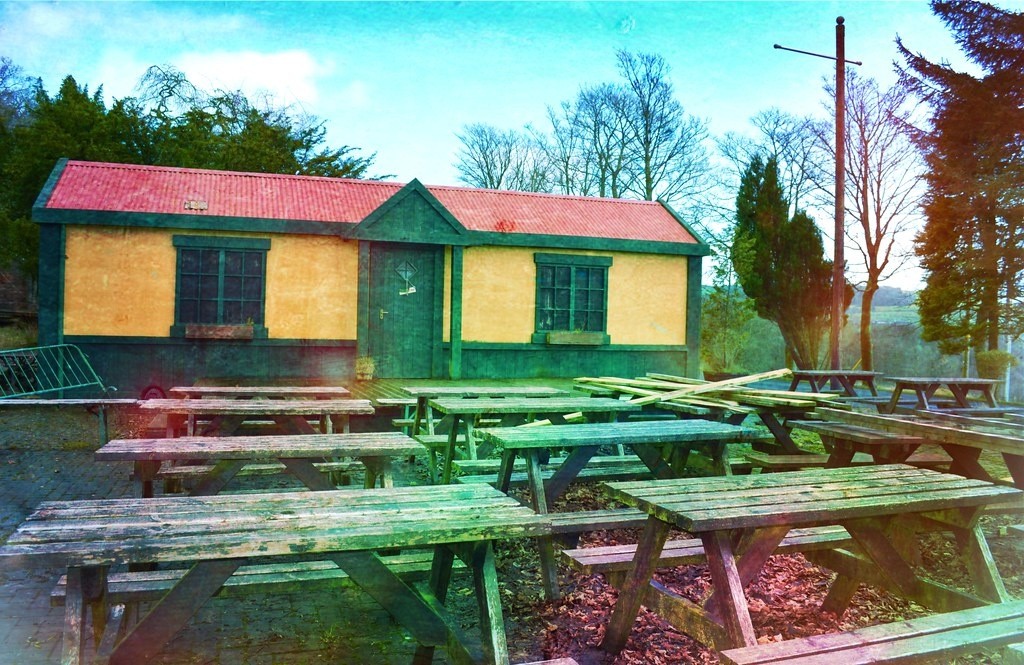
[471,418,760,602]
[0,483,552,665]
[169,386,352,436]
[571,371,1024,486]
[601,463,1024,655]
[428,398,641,486]
[399,386,570,485]
[139,398,376,497]
[94,431,426,572]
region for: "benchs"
[52,397,1024,665]
